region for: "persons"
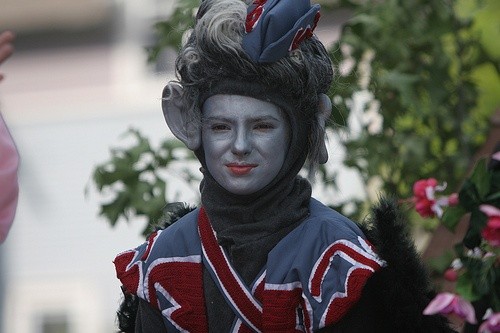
[111,1,458,333]
